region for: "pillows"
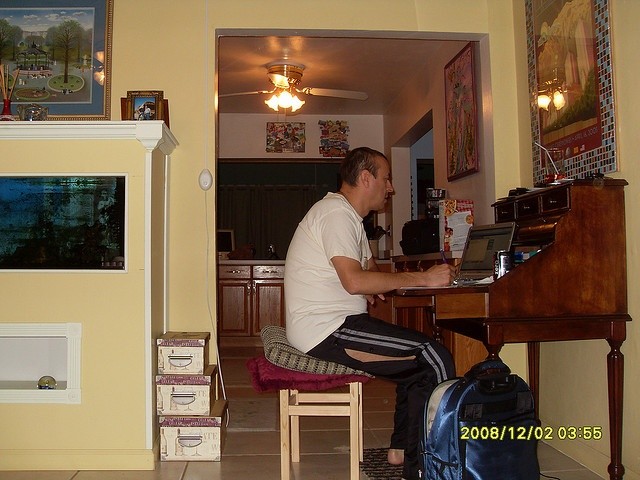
[245,353,368,391]
[260,325,375,378]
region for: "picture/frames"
[1,172,129,272]
[444,40,480,182]
[525,0,620,187]
[120,97,169,129]
[0,0,115,120]
[127,90,163,119]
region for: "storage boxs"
[157,399,229,462]
[156,331,211,375]
[155,364,219,415]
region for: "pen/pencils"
[439,248,447,263]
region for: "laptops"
[454,222,515,288]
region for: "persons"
[144,105,152,120]
[284,146,456,480]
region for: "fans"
[218,60,369,101]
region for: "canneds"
[492,249,513,281]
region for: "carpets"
[348,448,404,480]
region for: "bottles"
[0,99,16,121]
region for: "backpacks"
[421,360,540,478]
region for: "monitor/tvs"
[216,229,236,260]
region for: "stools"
[280,382,363,480]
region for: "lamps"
[264,88,306,113]
[537,77,566,111]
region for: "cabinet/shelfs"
[395,259,490,377]
[386,178,632,480]
[219,265,285,347]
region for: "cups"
[368,240,379,258]
